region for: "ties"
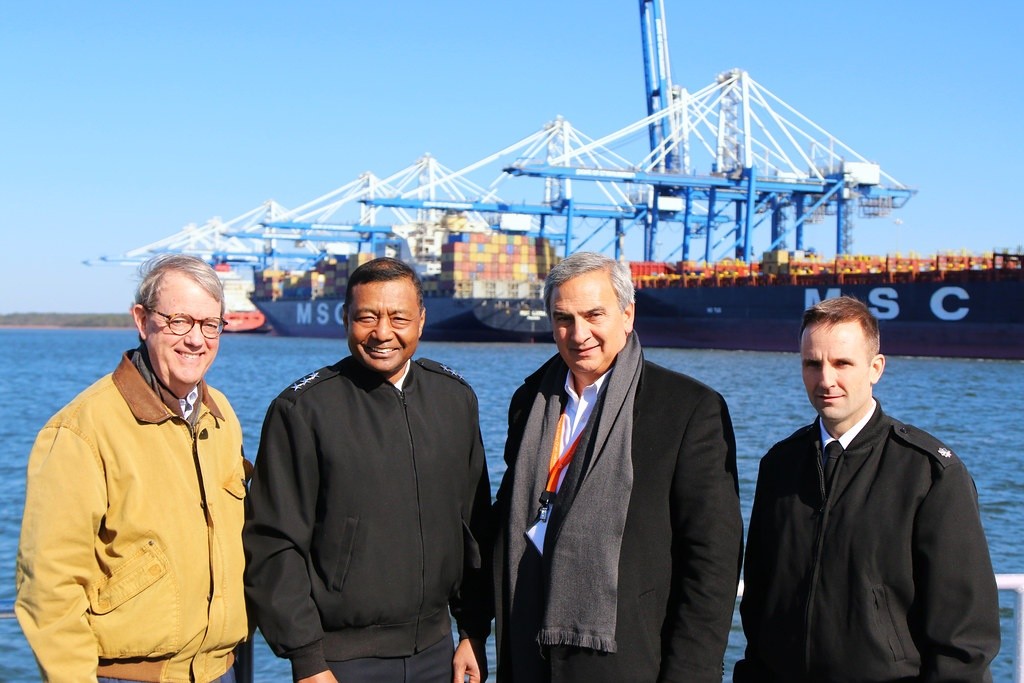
[824,439,844,490]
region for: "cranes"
[66,1,920,267]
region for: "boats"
[213,262,266,334]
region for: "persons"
[245,257,495,683]
[13,254,253,683]
[491,252,744,683]
[733,296,1001,683]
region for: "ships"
[248,214,1024,359]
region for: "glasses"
[145,305,228,340]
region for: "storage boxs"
[647,192,683,212]
[840,161,880,186]
[253,213,562,303]
[776,170,809,184]
[764,250,788,274]
